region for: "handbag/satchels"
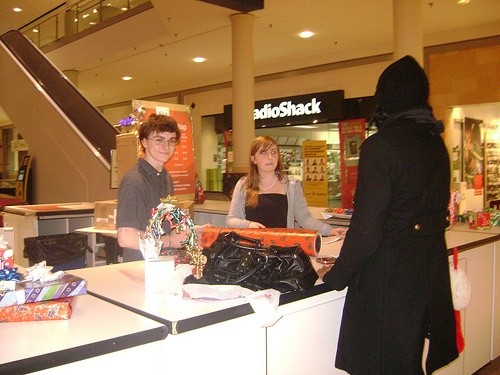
[183,231,319,294]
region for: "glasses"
[147,138,179,146]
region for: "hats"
[373,55,429,113]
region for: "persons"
[115,113,212,263]
[225,135,348,239]
[316,53,459,375]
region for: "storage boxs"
[95,200,118,230]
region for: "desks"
[5,202,95,267]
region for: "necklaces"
[258,174,278,190]
